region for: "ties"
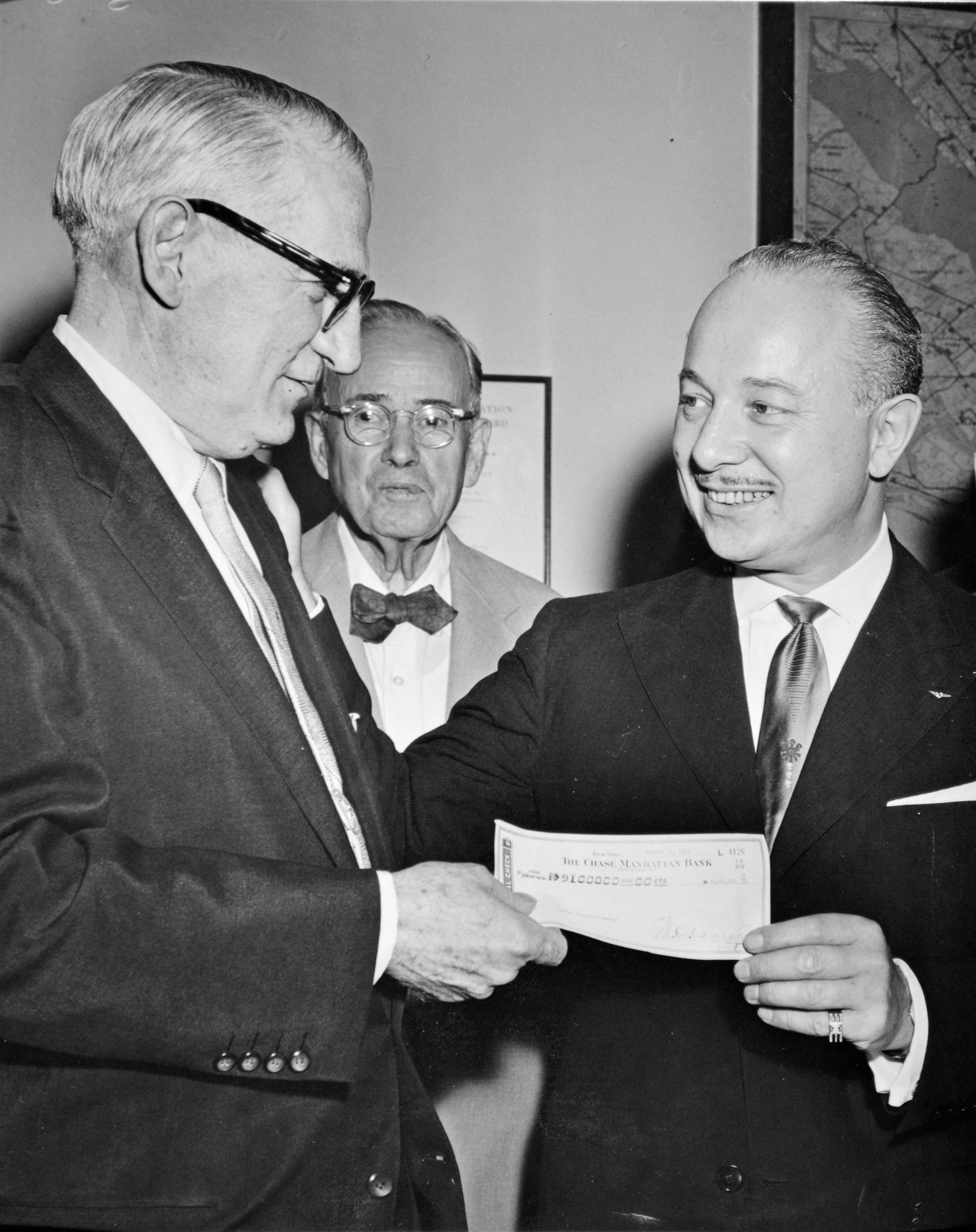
[755,595,835,856]
[191,455,374,871]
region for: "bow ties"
[349,584,458,643]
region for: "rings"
[829,1010,843,1043]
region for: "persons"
[301,298,561,1232]
[252,238,976,1232]
[0,60,567,1232]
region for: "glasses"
[316,402,480,448]
[184,198,376,332]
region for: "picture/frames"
[447,375,552,588]
[757,1,976,562]
